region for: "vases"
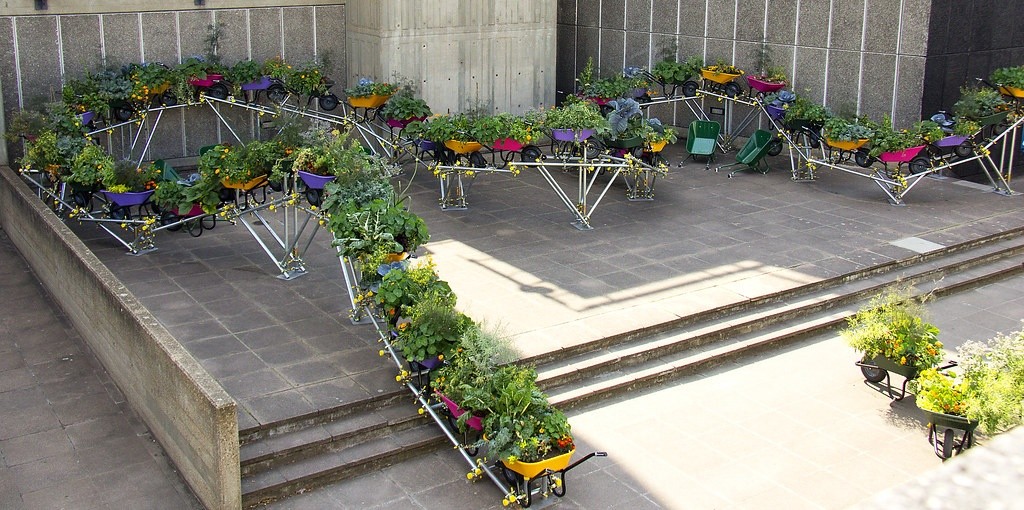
[629,88,645,97]
[482,432,576,479]
[346,94,388,109]
[867,352,915,378]
[735,129,773,167]
[685,120,721,155]
[792,117,825,133]
[880,144,926,162]
[927,411,979,432]
[550,127,595,141]
[605,136,643,149]
[936,135,969,147]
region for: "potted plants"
[952,82,1011,126]
[150,176,220,218]
[173,19,230,87]
[129,61,177,94]
[444,113,487,154]
[381,88,433,127]
[651,36,703,86]
[606,98,644,149]
[331,197,431,262]
[575,56,617,105]
[764,90,796,119]
[2,106,56,144]
[230,57,272,90]
[391,290,475,372]
[14,128,86,175]
[988,65,1024,97]
[644,117,679,153]
[746,43,790,92]
[700,59,745,84]
[822,116,873,150]
[66,146,108,199]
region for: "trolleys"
[15,58,1024,509]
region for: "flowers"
[0,54,574,464]
[907,366,972,416]
[539,93,602,129]
[839,269,946,373]
[617,65,982,157]
